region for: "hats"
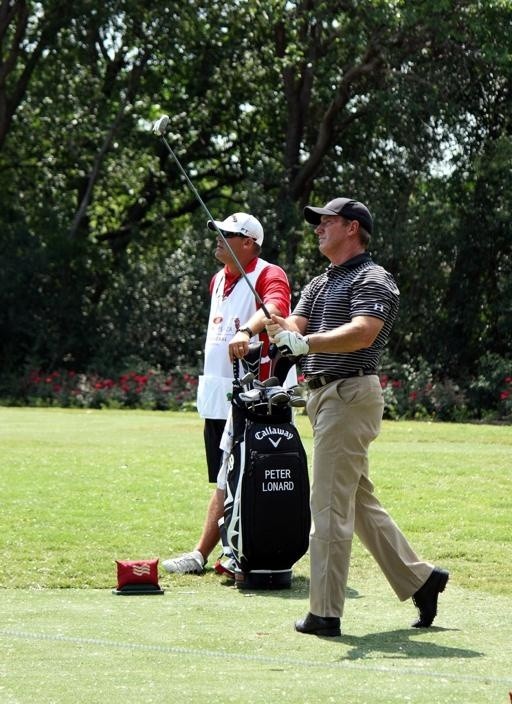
[207,211,264,247]
[304,197,374,234]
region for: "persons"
[262,195,451,637]
[156,210,294,578]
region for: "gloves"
[275,329,310,358]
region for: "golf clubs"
[153,115,273,321]
[239,372,307,414]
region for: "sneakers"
[163,550,236,574]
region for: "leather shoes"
[293,612,342,638]
[411,567,449,628]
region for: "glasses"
[220,229,249,239]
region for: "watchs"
[235,325,253,338]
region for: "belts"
[309,368,380,390]
